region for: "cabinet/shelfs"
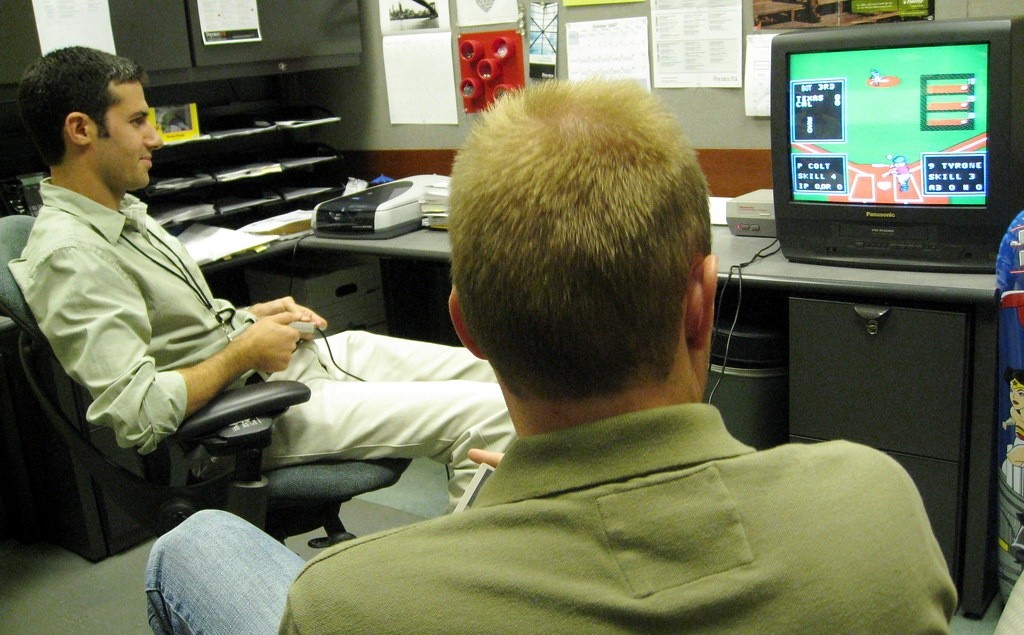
[790,297,973,587]
[0,0,362,103]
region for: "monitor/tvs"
[770,18,1024,274]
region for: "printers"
[310,175,435,240]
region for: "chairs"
[0,216,413,551]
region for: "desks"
[0,180,1007,620]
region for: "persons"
[144,77,957,635]
[5,45,518,516]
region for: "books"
[152,116,449,235]
